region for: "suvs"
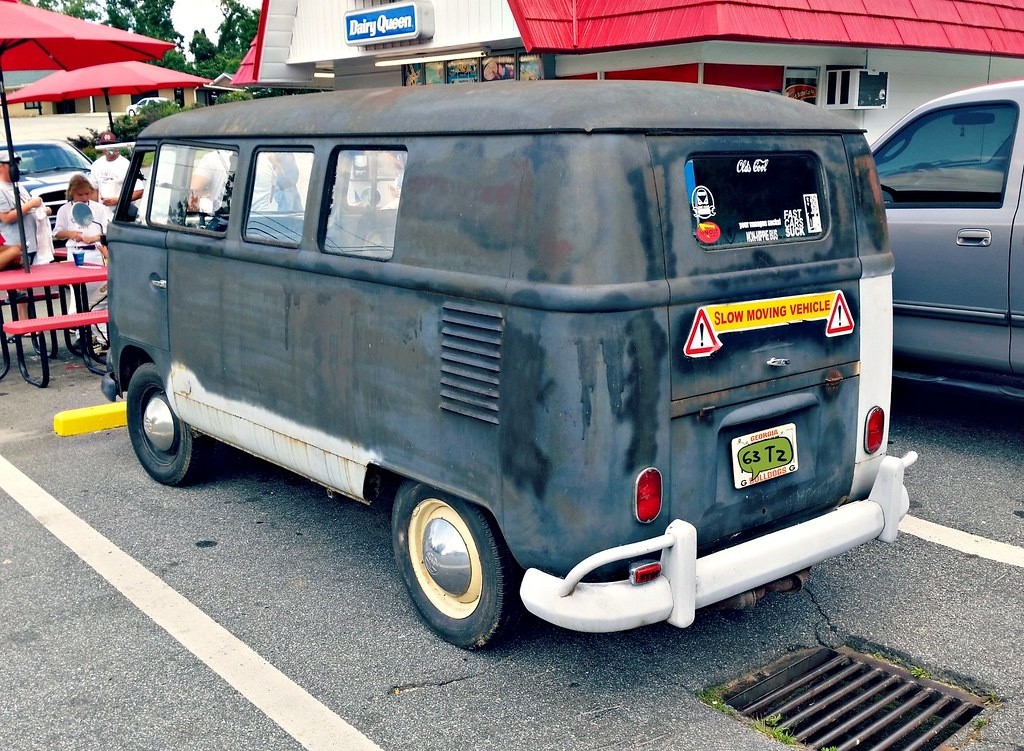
[868,77,1024,409]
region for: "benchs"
[2,309,109,388]
[0,290,61,358]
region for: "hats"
[99,131,118,144]
[1,149,21,163]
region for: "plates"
[483,62,497,80]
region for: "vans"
[93,80,920,652]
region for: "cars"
[0,138,96,233]
[124,97,180,119]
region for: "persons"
[190,145,303,213]
[0,132,145,337]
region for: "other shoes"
[91,333,108,356]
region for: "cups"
[72,252,85,265]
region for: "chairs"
[35,157,50,169]
[354,209,396,259]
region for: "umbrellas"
[2,0,177,352]
[6,60,216,135]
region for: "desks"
[0,261,110,380]
